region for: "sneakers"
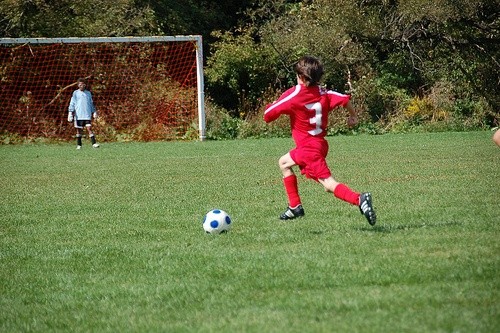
[359,192,377,226]
[280,204,305,220]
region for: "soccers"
[202,209,231,235]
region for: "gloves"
[93,113,97,119]
[67,112,73,122]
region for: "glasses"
[79,84,85,87]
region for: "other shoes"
[92,144,99,148]
[77,146,82,150]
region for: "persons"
[67,78,100,149]
[263,56,376,226]
